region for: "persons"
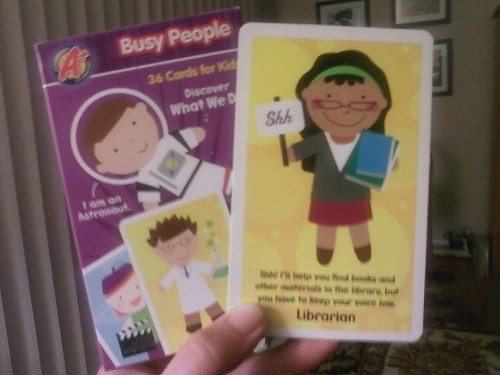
[95,301,344,375]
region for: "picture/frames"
[314,1,371,27]
[430,37,454,97]
[390,1,452,29]
